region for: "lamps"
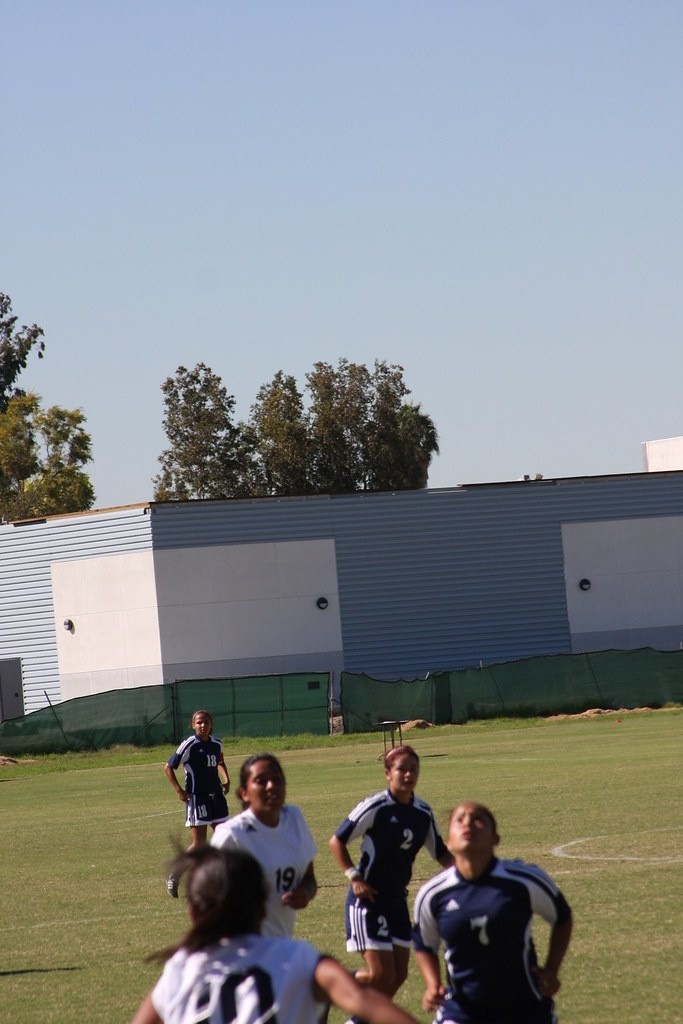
[580,579,591,590]
[64,619,72,630]
[317,598,328,609]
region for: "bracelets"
[345,867,360,880]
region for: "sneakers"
[165,872,178,898]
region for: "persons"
[328,746,456,1024]
[132,850,419,1024]
[209,753,318,939]
[164,710,230,898]
[413,802,572,1024]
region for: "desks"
[376,720,408,761]
